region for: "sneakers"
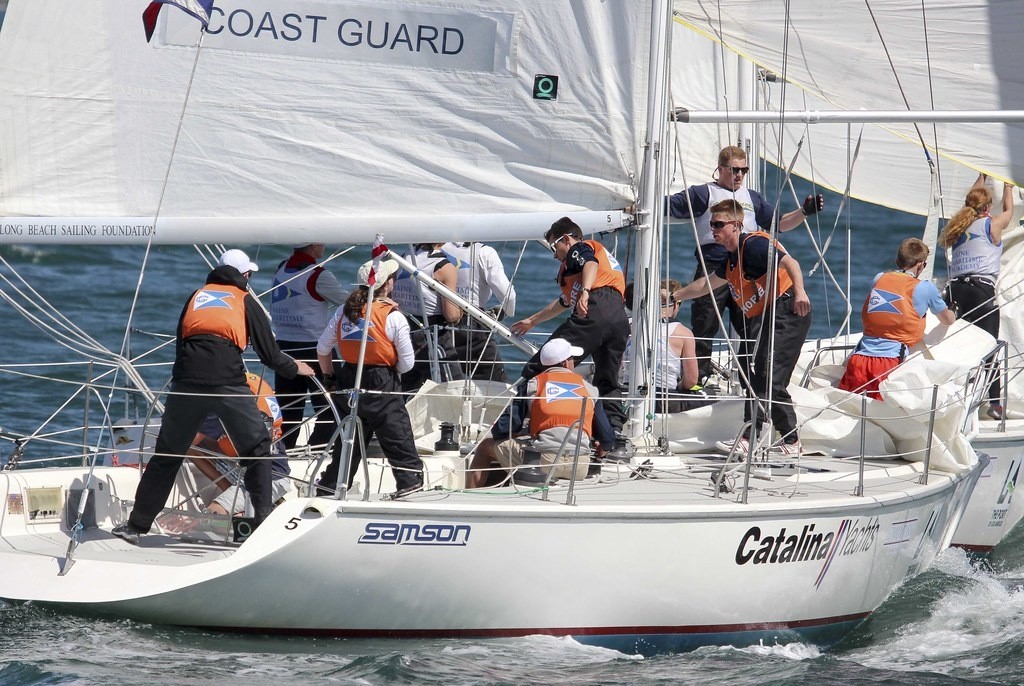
[773,440,802,455]
[714,435,763,457]
[986,403,1009,420]
[112,520,139,544]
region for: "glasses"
[677,300,681,306]
[242,271,252,278]
[709,221,744,228]
[550,233,572,254]
[722,162,749,174]
[920,259,927,268]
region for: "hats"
[357,259,398,293]
[218,249,259,276]
[540,338,584,366]
[290,243,309,249]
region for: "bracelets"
[670,292,677,303]
[581,288,590,293]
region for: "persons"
[838,238,956,402]
[185,372,291,519]
[111,249,316,544]
[387,243,464,388]
[466,338,615,489]
[939,172,1015,419]
[508,217,631,434]
[269,243,351,451]
[441,242,516,382]
[316,259,424,496]
[661,198,812,458]
[621,146,823,391]
[618,279,698,413]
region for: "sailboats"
[0,0,1024,644]
